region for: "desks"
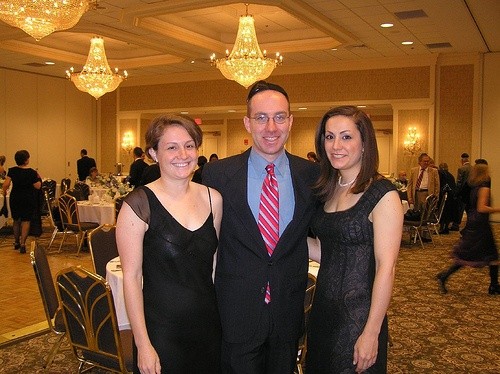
[76,201,116,225]
[90,188,119,201]
[0,190,14,232]
[105,257,144,331]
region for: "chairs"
[404,194,438,251]
[73,183,91,201]
[61,178,71,196]
[30,241,68,370]
[89,224,120,278]
[44,191,83,256]
[58,194,100,257]
[409,192,448,248]
[41,179,57,228]
[53,264,135,374]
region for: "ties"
[256,165,280,305]
[415,169,425,190]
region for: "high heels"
[435,272,448,294]
[489,284,500,295]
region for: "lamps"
[402,126,422,156]
[0,0,100,42]
[71,36,127,101]
[212,4,283,90]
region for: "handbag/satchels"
[0,195,9,218]
[405,209,422,221]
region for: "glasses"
[249,114,290,125]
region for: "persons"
[0,156,6,187]
[115,114,223,374]
[191,156,208,183]
[307,152,318,164]
[1,150,42,254]
[76,149,98,184]
[307,105,404,374]
[437,162,456,235]
[449,153,490,234]
[396,171,408,185]
[124,146,161,186]
[208,154,218,162]
[199,80,327,374]
[437,164,500,295]
[407,153,439,240]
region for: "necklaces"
[338,173,360,188]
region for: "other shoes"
[20,247,26,253]
[434,228,449,235]
[450,224,459,230]
[412,237,432,242]
[14,243,21,250]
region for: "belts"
[417,189,428,191]
